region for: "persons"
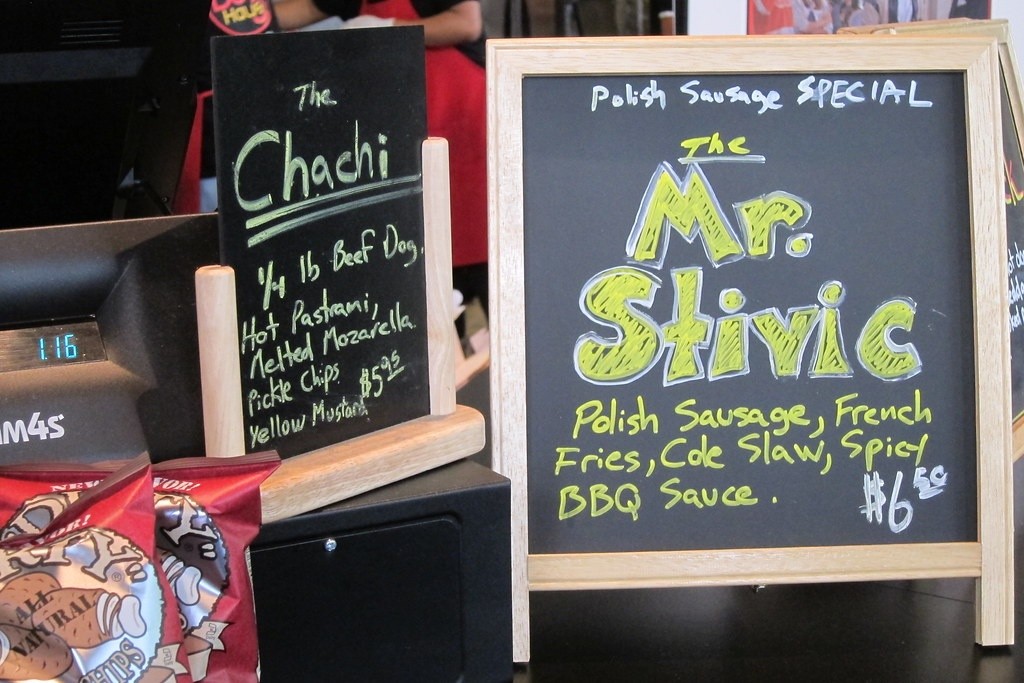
[791,0,988,35]
[271,0,489,342]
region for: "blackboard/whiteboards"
[481,33,1014,592]
[193,23,461,467]
